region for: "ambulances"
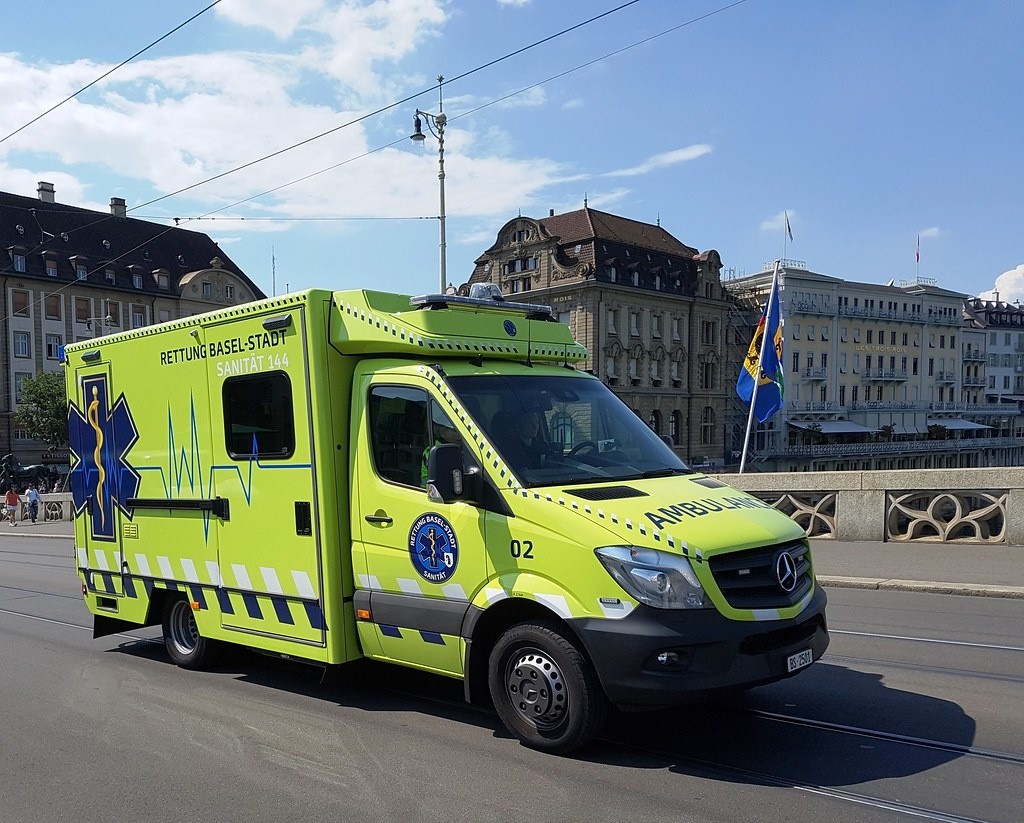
[58,284,832,751]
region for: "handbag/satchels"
[30,500,38,506]
[1,505,7,515]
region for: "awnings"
[785,417,994,434]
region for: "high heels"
[9,522,18,526]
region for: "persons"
[508,408,554,470]
[5,480,64,527]
[420,425,466,489]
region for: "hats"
[29,483,32,485]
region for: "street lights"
[413,75,447,293]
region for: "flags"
[785,212,794,243]
[736,275,784,424]
[916,234,919,263]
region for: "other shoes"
[32,519,38,524]
